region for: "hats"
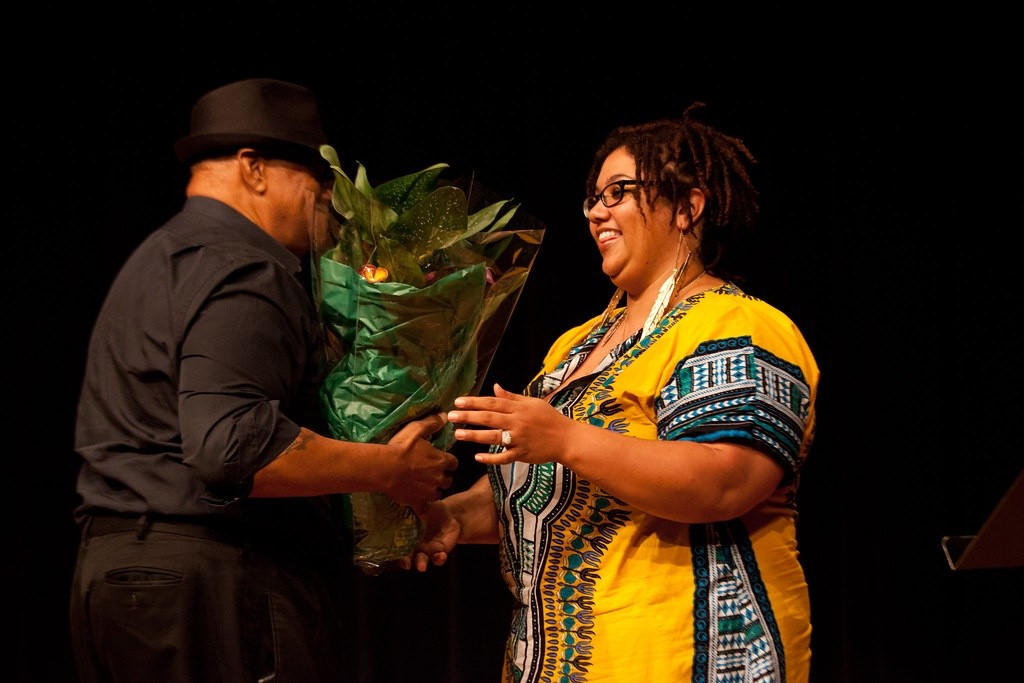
[173,78,348,176]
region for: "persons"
[395,100,821,683]
[66,76,459,682]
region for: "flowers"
[307,144,547,572]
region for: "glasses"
[582,180,686,218]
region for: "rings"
[501,429,512,446]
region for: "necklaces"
[677,271,708,297]
